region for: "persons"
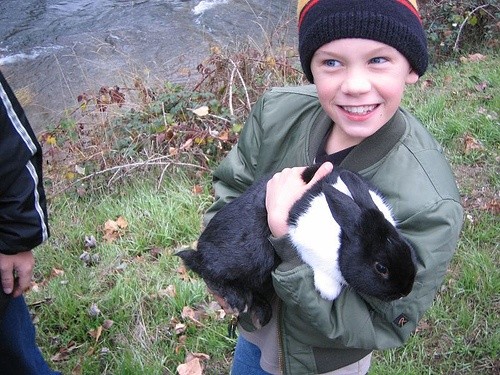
[201,1,465,375]
[0,72,67,375]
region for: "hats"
[295,0,429,84]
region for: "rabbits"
[173,166,418,333]
